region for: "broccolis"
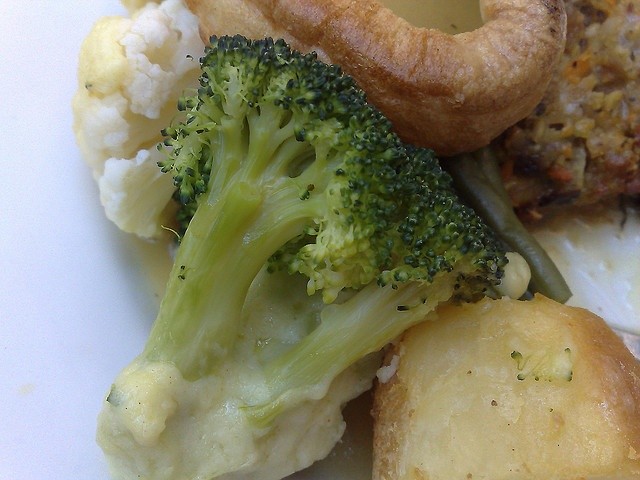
[75,0,508,479]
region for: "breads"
[372,292,640,480]
